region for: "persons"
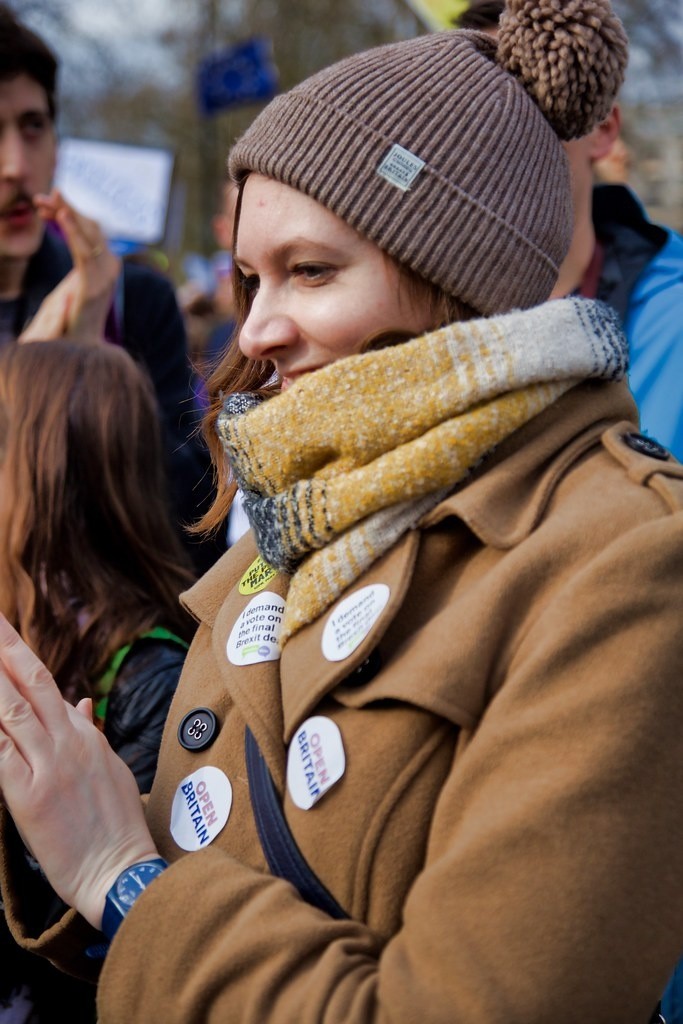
[0,0,683,1024]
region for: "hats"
[229,0,629,316]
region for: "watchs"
[85,858,170,958]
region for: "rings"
[92,246,102,256]
